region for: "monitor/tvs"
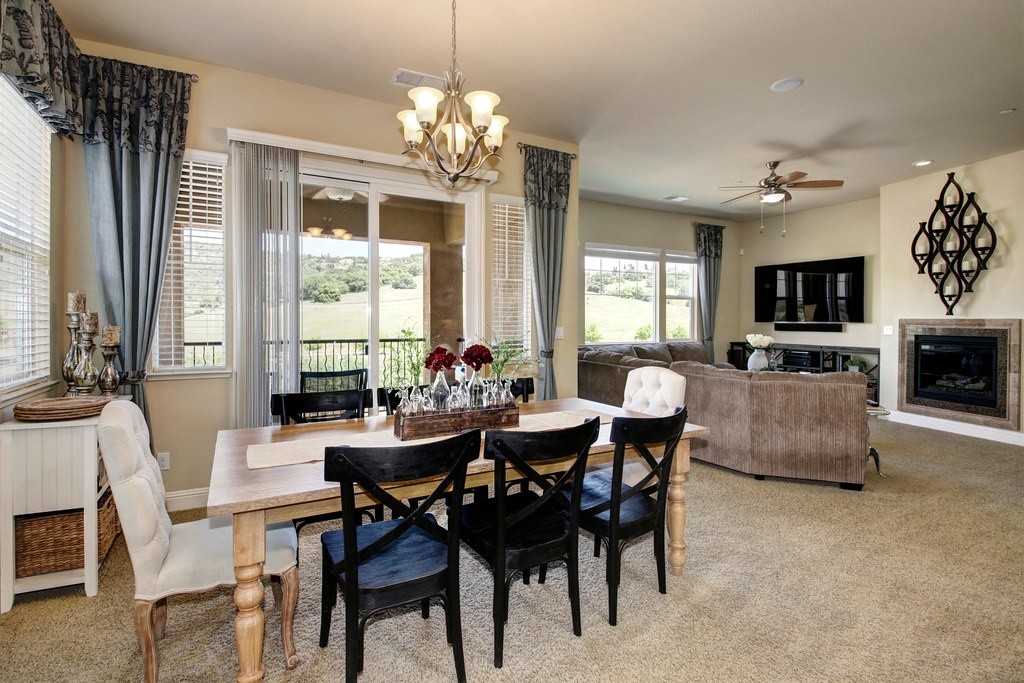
[754,256,865,323]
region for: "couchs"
[577,341,869,491]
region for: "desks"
[207,397,710,683]
[866,404,891,478]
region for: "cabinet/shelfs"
[730,342,880,406]
[0,394,133,613]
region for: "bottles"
[447,386,461,408]
[421,387,431,412]
[770,354,777,370]
[62,312,121,397]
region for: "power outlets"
[155,453,169,470]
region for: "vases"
[747,349,768,371]
[431,367,488,414]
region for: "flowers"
[424,346,494,372]
[745,334,777,353]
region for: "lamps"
[302,220,353,240]
[394,0,509,188]
[760,187,785,203]
[325,187,354,203]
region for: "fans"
[311,186,389,203]
[716,161,845,237]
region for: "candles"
[918,196,988,295]
[68,290,120,344]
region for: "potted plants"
[475,323,546,409]
[380,317,441,416]
[844,359,867,372]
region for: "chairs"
[94,365,687,683]
